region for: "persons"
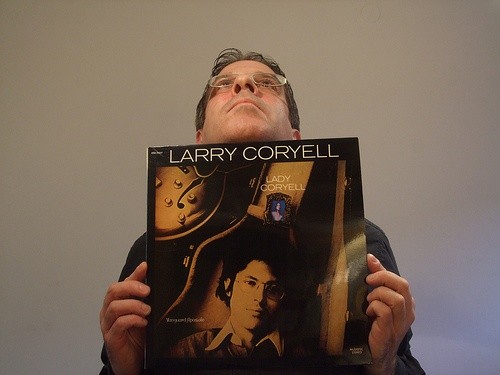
[167,235,293,362]
[98,48,425,375]
[271,201,283,221]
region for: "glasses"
[205,73,288,101]
[234,278,288,301]
